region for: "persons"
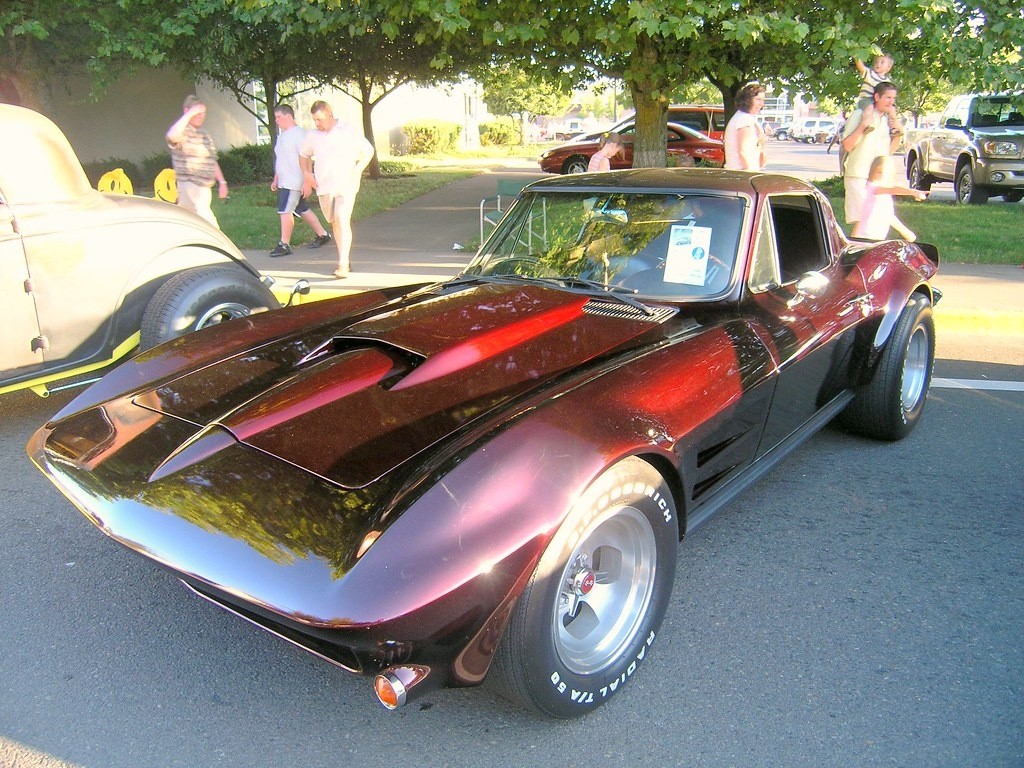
[853,52,904,136]
[827,111,847,175]
[166,94,228,228]
[724,82,765,169]
[842,82,904,237]
[584,132,622,212]
[270,104,331,256]
[300,100,374,279]
[850,155,931,242]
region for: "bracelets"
[218,181,226,184]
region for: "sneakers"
[308,234,332,249]
[269,241,292,257]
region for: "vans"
[792,117,837,143]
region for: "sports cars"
[28,165,943,720]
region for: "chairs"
[476,177,547,255]
[973,110,1023,127]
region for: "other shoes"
[333,262,351,278]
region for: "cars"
[760,122,785,134]
[0,98,310,398]
[538,122,727,174]
[571,105,764,168]
[904,93,1024,205]
[771,125,790,141]
